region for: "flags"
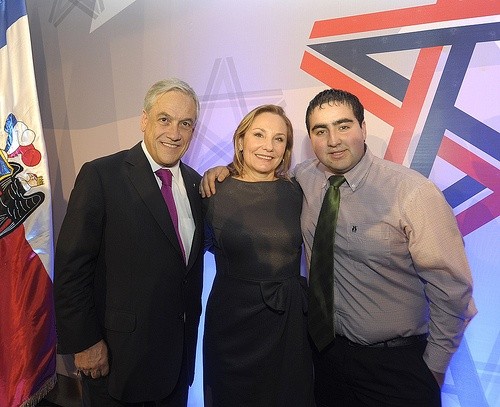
[0,0,55,407]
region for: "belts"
[371,333,432,350]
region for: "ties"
[306,175,348,352]
[154,168,188,260]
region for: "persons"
[198,89,479,407]
[203,103,313,407]
[54,80,204,407]
